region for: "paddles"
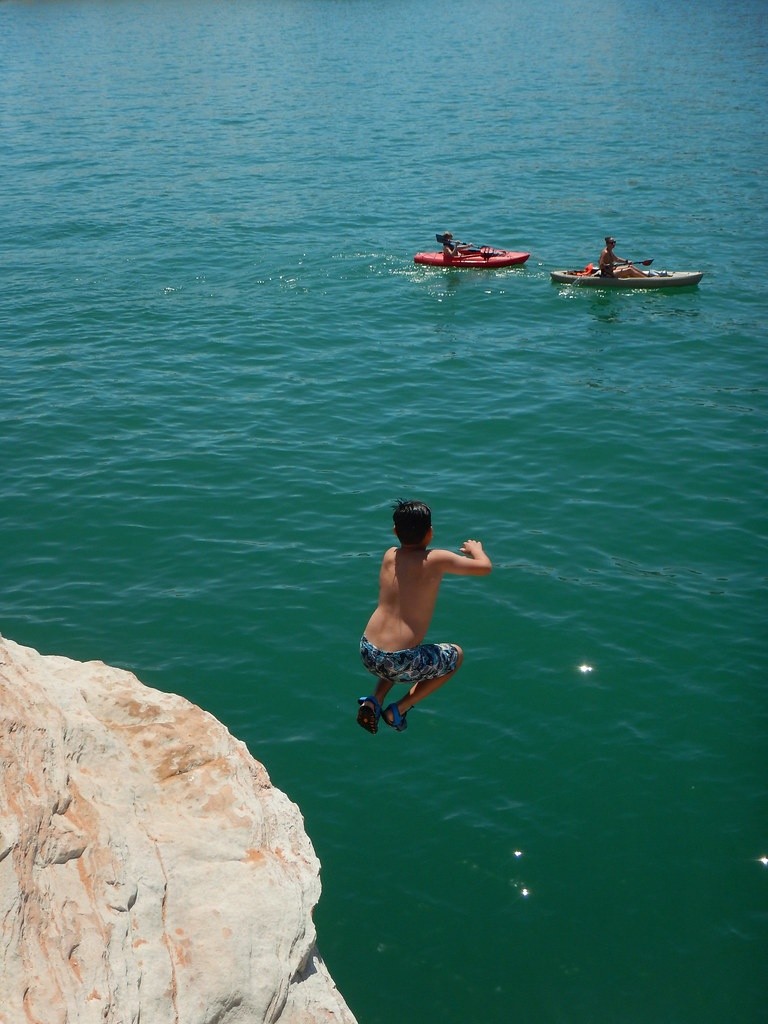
[603,259,654,268]
[435,233,481,249]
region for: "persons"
[355,496,493,736]
[599,236,649,278]
[442,232,474,258]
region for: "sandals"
[356,696,384,735]
[380,701,409,731]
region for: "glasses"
[608,241,616,244]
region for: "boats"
[413,245,531,267]
[548,270,705,288]
